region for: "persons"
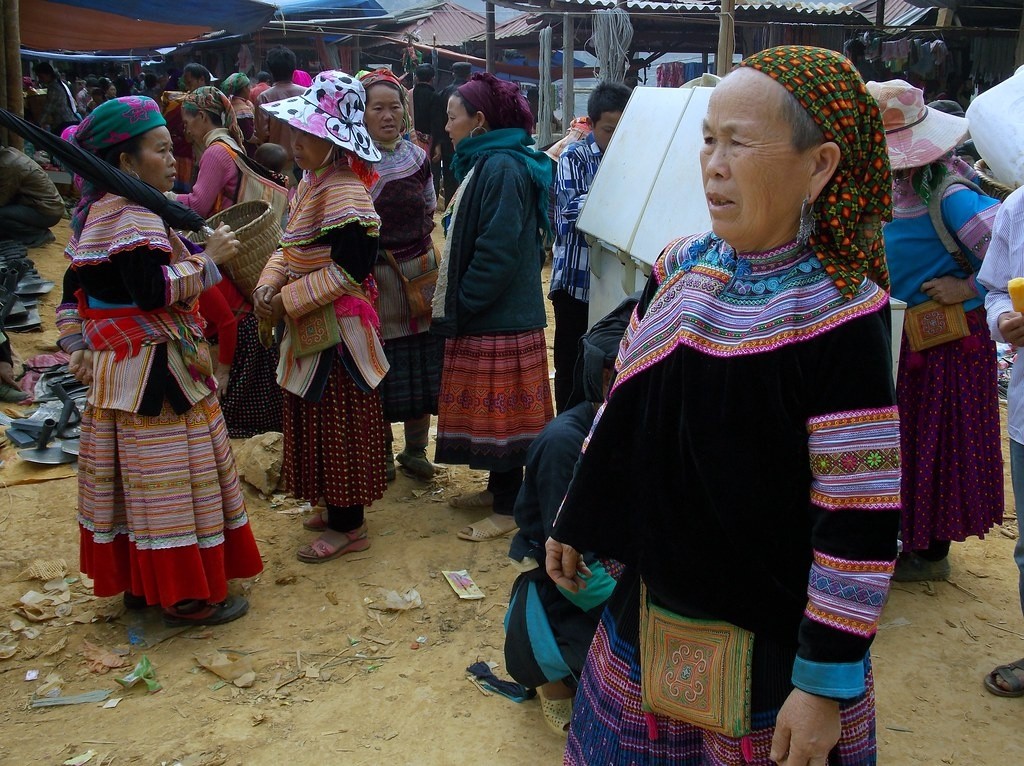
[0,144,65,247]
[54,96,266,623]
[155,43,314,229]
[543,46,901,766]
[22,76,40,95]
[354,63,443,483]
[50,65,176,121]
[551,81,632,415]
[160,84,286,440]
[863,65,1024,702]
[253,71,391,564]
[514,293,647,737]
[400,62,474,211]
[33,60,82,135]
[432,73,555,539]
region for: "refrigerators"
[573,86,908,393]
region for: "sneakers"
[384,453,396,482]
[163,593,249,629]
[121,591,165,615]
[396,451,434,478]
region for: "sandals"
[984,656,1024,698]
[297,519,370,563]
[303,511,368,533]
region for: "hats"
[209,72,219,82]
[865,79,971,170]
[259,69,385,162]
[928,100,965,118]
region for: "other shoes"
[25,230,55,248]
[0,387,30,402]
[891,550,952,583]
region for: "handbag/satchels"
[287,303,344,368]
[407,268,441,331]
[636,603,755,762]
[180,335,219,395]
[902,298,969,352]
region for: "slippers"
[536,683,575,737]
[457,516,520,541]
[449,493,487,510]
